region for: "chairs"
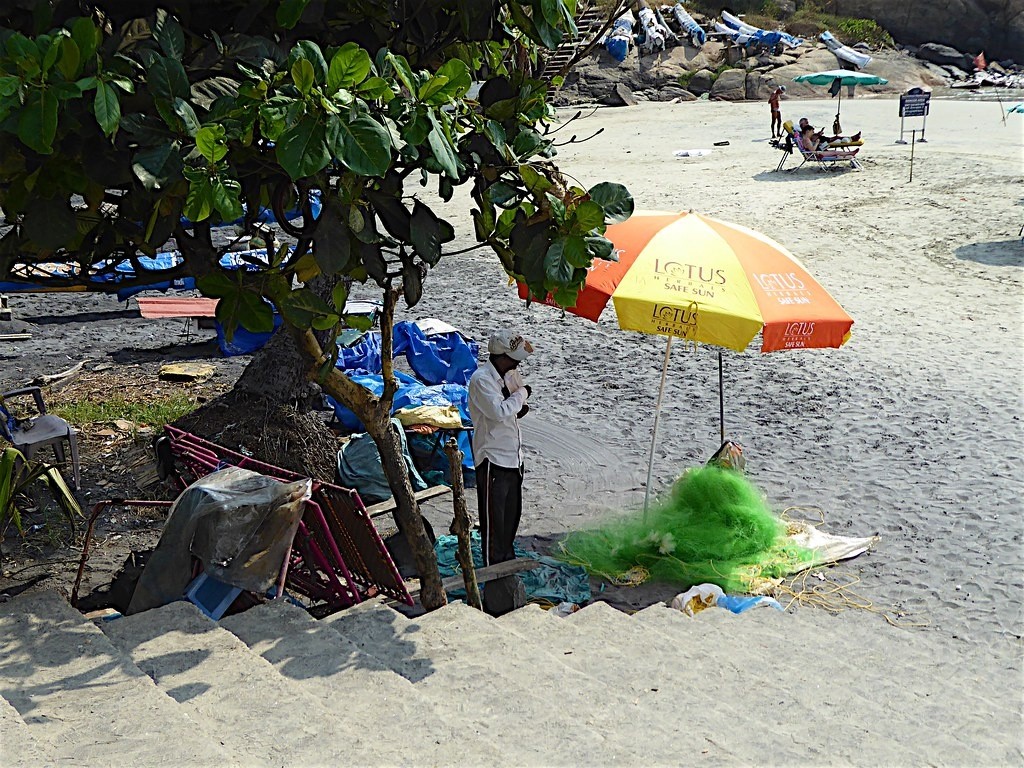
[790,136,865,174]
[776,119,865,163]
[0,385,80,496]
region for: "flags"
[974,52,986,71]
[828,78,841,98]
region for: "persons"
[468,330,532,617]
[768,85,786,137]
[799,118,861,144]
[802,125,859,157]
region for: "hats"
[487,329,534,360]
[779,85,786,93]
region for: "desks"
[136,295,219,352]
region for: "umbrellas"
[791,70,888,163]
[134,190,325,228]
[950,67,1024,127]
[1006,102,1024,236]
[516,208,853,525]
